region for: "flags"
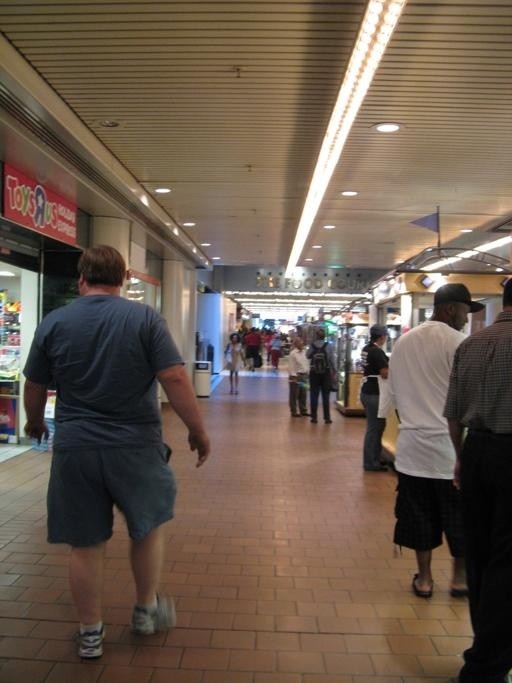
[401,204,448,240]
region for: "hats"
[369,322,390,336]
[433,282,485,313]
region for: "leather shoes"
[365,466,389,473]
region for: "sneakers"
[72,624,106,660]
[130,589,178,636]
[410,572,434,597]
[447,588,471,600]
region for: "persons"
[287,337,311,418]
[224,325,283,395]
[359,323,390,471]
[442,274,512,683]
[305,328,336,424]
[387,283,485,599]
[23,244,211,659]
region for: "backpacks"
[310,342,329,375]
